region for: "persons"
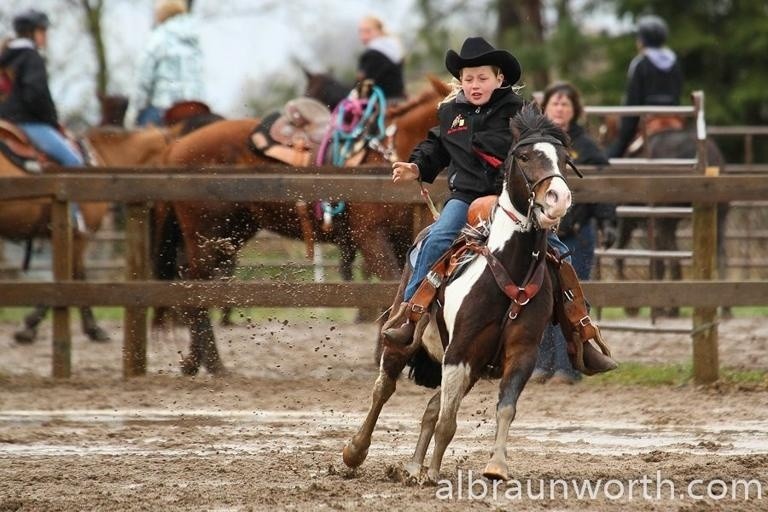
[606,15,683,157]
[358,16,407,108]
[119,0,206,127]
[531,83,619,391]
[383,36,618,373]
[0,11,85,238]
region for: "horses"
[0,110,226,350]
[594,107,731,324]
[294,63,357,285]
[337,97,576,498]
[148,73,458,382]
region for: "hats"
[12,8,51,30]
[443,35,523,89]
[631,15,672,48]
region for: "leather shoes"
[381,322,416,347]
[563,328,620,376]
